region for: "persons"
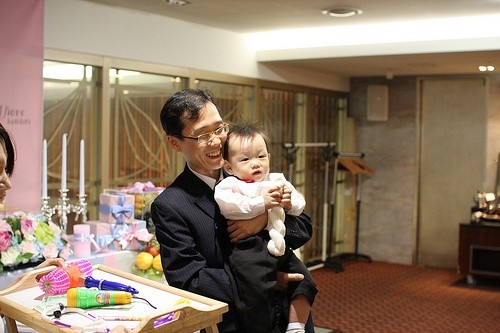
[214,117,318,333]
[151,89,316,333]
[0,122,65,333]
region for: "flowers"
[0,209,71,273]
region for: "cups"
[73,224,91,258]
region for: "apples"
[145,245,158,257]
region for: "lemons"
[135,252,163,271]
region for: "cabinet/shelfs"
[458,223,500,287]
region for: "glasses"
[174,122,229,144]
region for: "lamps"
[321,7,362,18]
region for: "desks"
[0,263,229,333]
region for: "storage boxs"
[86,182,166,252]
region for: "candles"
[40,133,87,199]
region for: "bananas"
[134,195,144,215]
